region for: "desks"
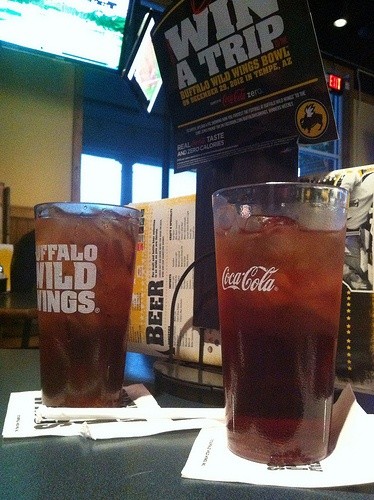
[0,347,374,500]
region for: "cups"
[212,181,348,467]
[33,202,141,408]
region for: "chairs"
[0,227,39,348]
